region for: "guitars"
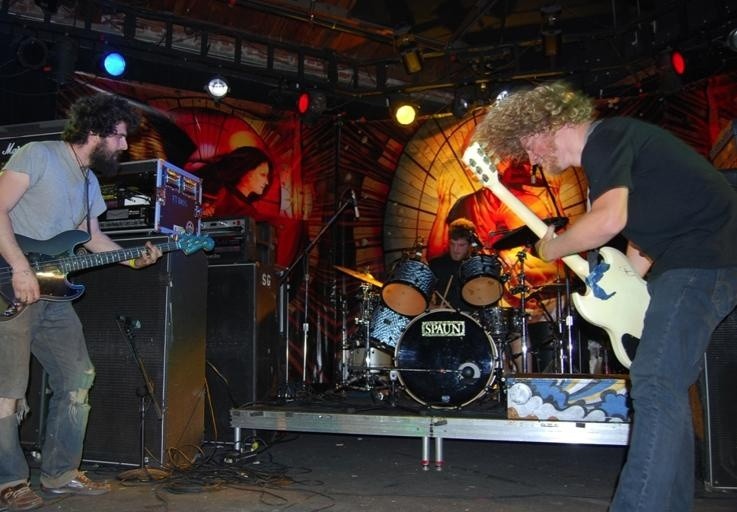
[0,231,216,322]
[460,141,651,368]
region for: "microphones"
[348,183,362,224]
[517,250,528,260]
[117,317,144,331]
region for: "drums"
[479,306,514,337]
[381,260,435,316]
[395,308,499,411]
[458,254,504,306]
[365,305,411,354]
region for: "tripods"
[481,338,512,404]
[536,289,581,373]
[337,308,392,392]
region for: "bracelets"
[125,255,140,269]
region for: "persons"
[471,78,737,510]
[429,219,481,310]
[203,147,273,222]
[0,90,163,511]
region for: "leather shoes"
[37,468,112,496]
[0,482,45,512]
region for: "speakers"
[17,352,53,448]
[205,260,287,446]
[76,236,210,471]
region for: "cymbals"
[492,216,568,250]
[532,283,572,289]
[333,263,383,288]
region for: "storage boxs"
[505,374,633,423]
